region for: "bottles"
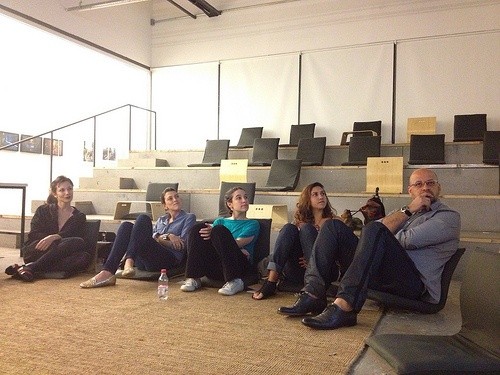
[158,269,169,299]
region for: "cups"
[95,258,104,273]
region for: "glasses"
[410,181,438,187]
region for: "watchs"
[400,205,412,217]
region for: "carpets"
[0,251,387,375]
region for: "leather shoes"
[302,303,357,327]
[278,292,327,315]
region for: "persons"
[181,187,260,296]
[252,182,340,300]
[80,187,196,288]
[4,175,89,282]
[276,167,461,330]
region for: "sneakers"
[180,280,198,291]
[218,279,244,295]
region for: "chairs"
[365,249,466,316]
[33,113,500,266]
[364,247,500,375]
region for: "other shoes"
[5,264,23,274]
[16,269,32,280]
[80,275,116,288]
[122,268,135,277]
[251,281,278,300]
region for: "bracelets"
[163,233,169,239]
